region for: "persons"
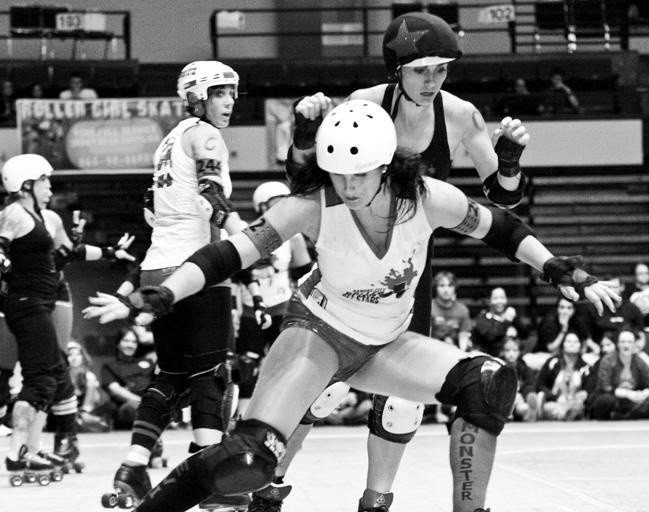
[0,80,16,127]
[248,12,531,512]
[0,189,87,446]
[113,59,274,506]
[59,76,98,101]
[503,78,531,116]
[0,152,136,474]
[82,100,624,512]
[537,74,580,117]
[219,180,315,486]
[28,81,45,98]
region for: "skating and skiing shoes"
[199,493,253,511]
[5,432,84,486]
[102,463,151,509]
[149,441,167,468]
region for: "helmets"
[177,60,240,107]
[315,99,397,176]
[1,153,54,195]
[252,181,292,213]
[383,11,461,83]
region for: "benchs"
[230,181,290,224]
[52,173,152,265]
[430,174,647,326]
